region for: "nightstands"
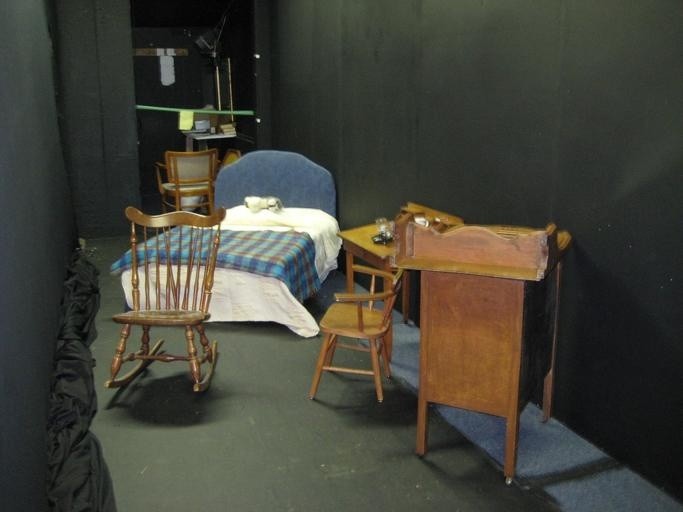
[393,210,573,486]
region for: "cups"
[375,217,391,246]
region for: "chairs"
[310,265,403,402]
[104,206,226,391]
[153,148,216,215]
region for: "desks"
[338,200,464,325]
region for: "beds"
[110,151,338,328]
